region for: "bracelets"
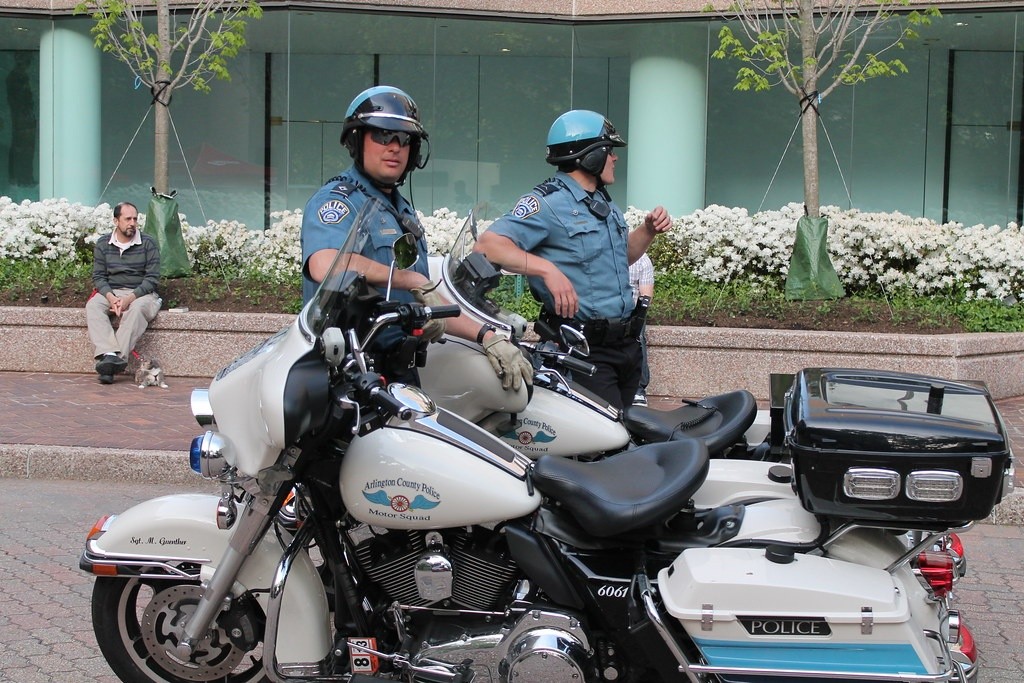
[524,252,528,276]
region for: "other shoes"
[99,375,113,384]
[95,355,127,375]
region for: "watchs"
[477,323,496,344]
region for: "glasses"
[370,128,413,147]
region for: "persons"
[628,254,654,409]
[86,203,162,384]
[472,110,671,414]
[300,85,535,397]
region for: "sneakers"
[632,394,647,407]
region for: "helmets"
[545,110,627,177]
[339,85,423,143]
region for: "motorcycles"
[78,193,1016,683]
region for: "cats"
[134,360,169,388]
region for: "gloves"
[412,279,445,344]
[482,335,533,392]
